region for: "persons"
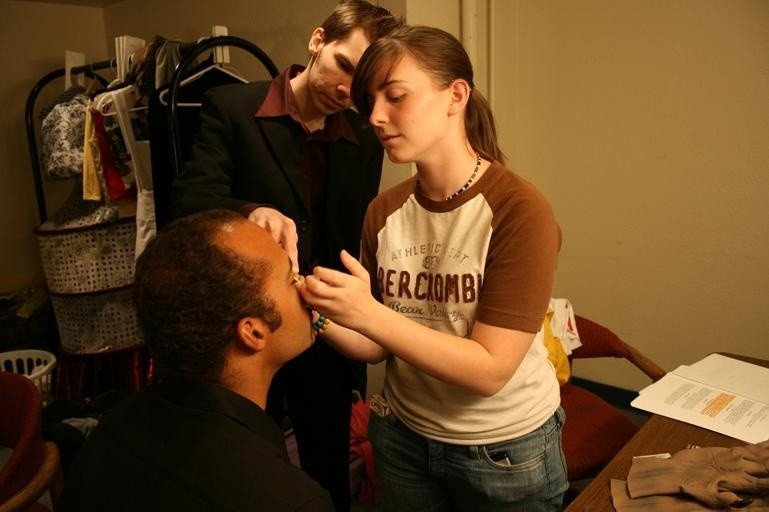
[292,22,571,511]
[70,208,334,510]
[170,0,403,510]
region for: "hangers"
[69,37,257,119]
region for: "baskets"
[0,349,57,407]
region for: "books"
[628,351,769,444]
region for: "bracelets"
[312,312,326,329]
[313,319,330,335]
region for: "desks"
[555,351,768,511]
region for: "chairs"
[555,312,668,496]
[1,372,66,511]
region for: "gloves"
[610,441,769,512]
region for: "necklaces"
[415,150,481,200]
